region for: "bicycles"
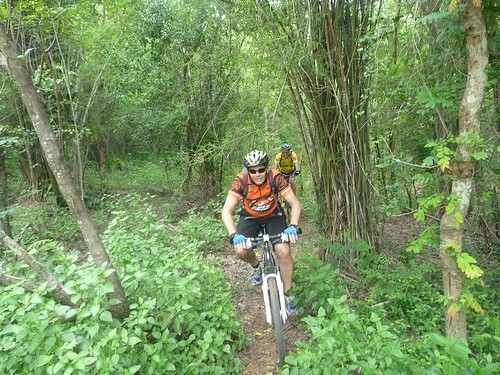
[280,170,301,216]
[231,222,302,369]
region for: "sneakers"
[284,294,298,315]
[251,266,263,286]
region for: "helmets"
[280,144,291,151]
[243,149,269,167]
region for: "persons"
[221,150,301,316]
[274,143,300,197]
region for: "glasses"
[249,167,266,174]
